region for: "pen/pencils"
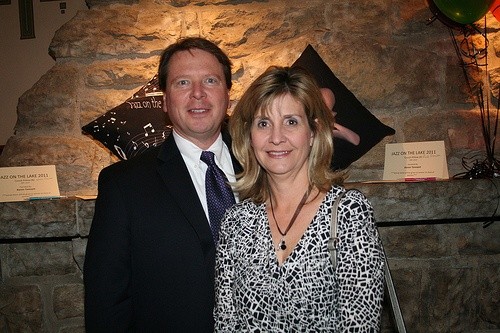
[401,177,443,181]
[29,195,67,200]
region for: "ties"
[201,151,236,248]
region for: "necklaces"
[268,180,315,251]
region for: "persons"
[214,65,385,333]
[83,37,250,333]
